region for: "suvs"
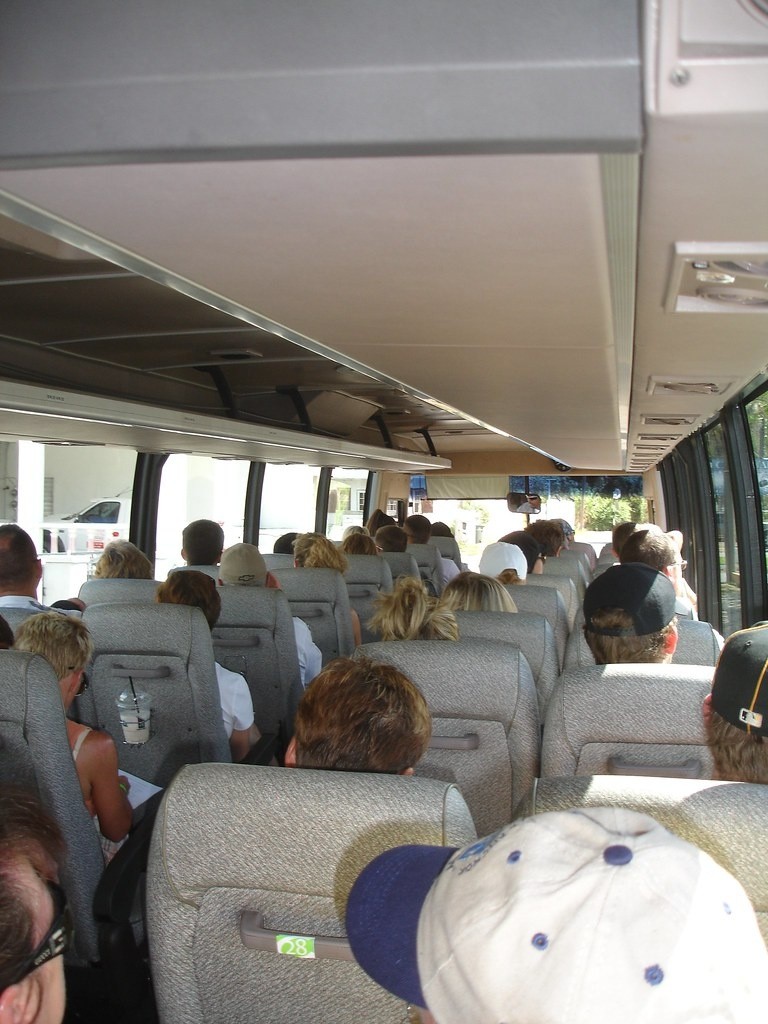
[43,496,132,554]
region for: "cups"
[116,688,153,744]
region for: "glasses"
[0,881,74,994]
[668,560,688,570]
[566,529,575,536]
[68,667,89,696]
[525,495,538,500]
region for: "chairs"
[0,535,768,1024]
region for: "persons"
[14,611,134,862]
[1,521,361,767]
[285,658,433,775]
[0,615,14,649]
[701,619,768,784]
[582,522,697,665]
[517,495,540,514]
[0,824,72,1024]
[344,808,768,1023]
[340,509,575,648]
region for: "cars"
[709,456,768,495]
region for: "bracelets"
[120,783,128,792]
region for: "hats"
[711,621,768,737]
[222,542,267,585]
[583,563,676,636]
[480,542,528,580]
[344,805,768,1024]
